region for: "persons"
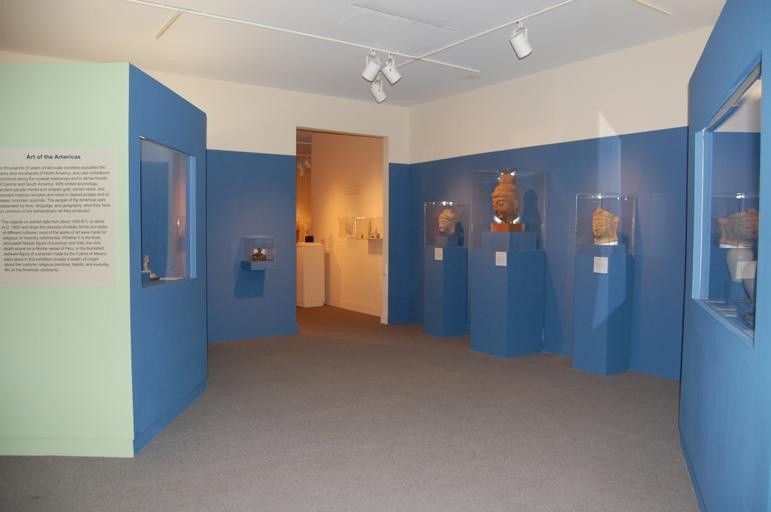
[438,209,456,239]
[592,209,619,244]
[253,247,267,263]
[303,219,312,236]
[492,175,521,225]
[718,209,758,249]
[143,255,156,279]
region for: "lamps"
[509,21,532,59]
[361,50,402,104]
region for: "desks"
[296,242,325,308]
